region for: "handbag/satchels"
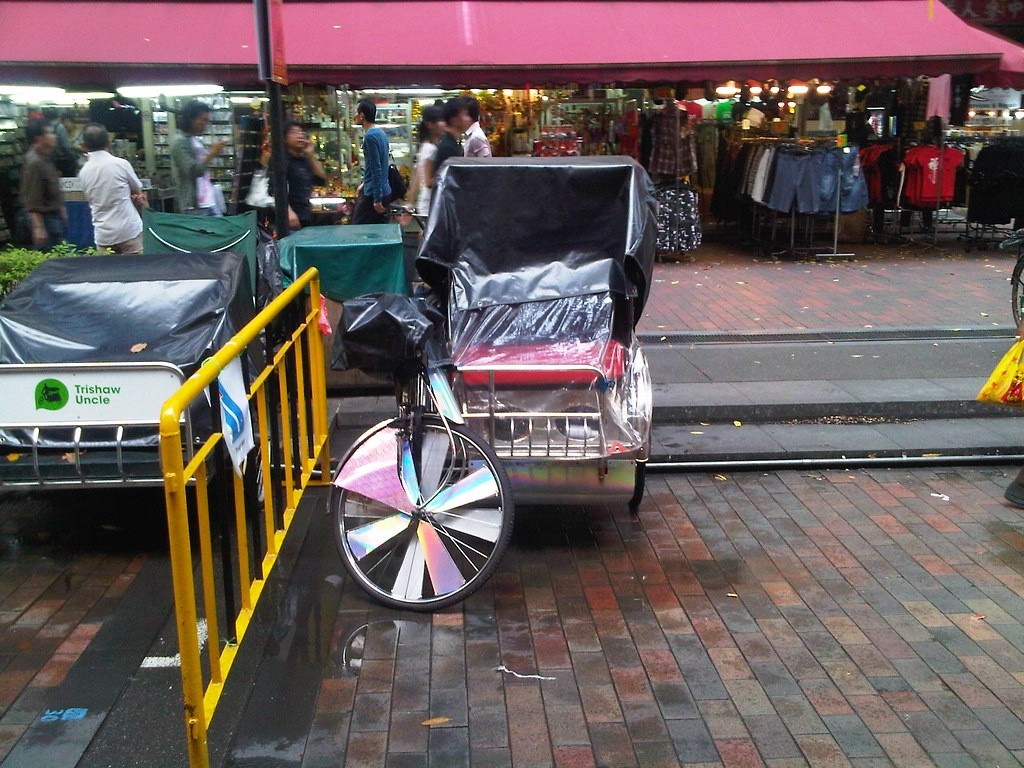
[245,170,290,208]
[382,165,406,208]
[976,339,1024,410]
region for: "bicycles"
[999,229,1024,326]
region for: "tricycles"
[327,156,655,612]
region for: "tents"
[0,1,1005,85]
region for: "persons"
[674,85,703,124]
[266,123,328,237]
[169,102,229,216]
[732,77,927,126]
[77,122,144,255]
[394,97,492,237]
[351,98,391,225]
[18,108,87,254]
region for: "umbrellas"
[963,26,1024,91]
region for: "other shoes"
[1005,467,1024,506]
